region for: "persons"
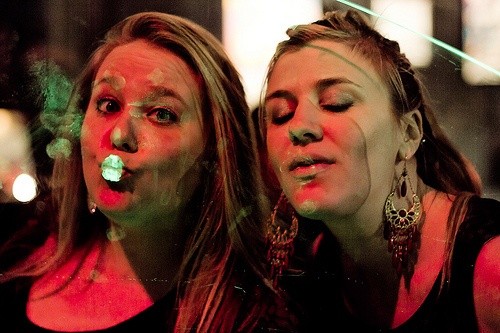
[260,11,500,333]
[0,13,283,333]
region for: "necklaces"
[338,253,383,294]
